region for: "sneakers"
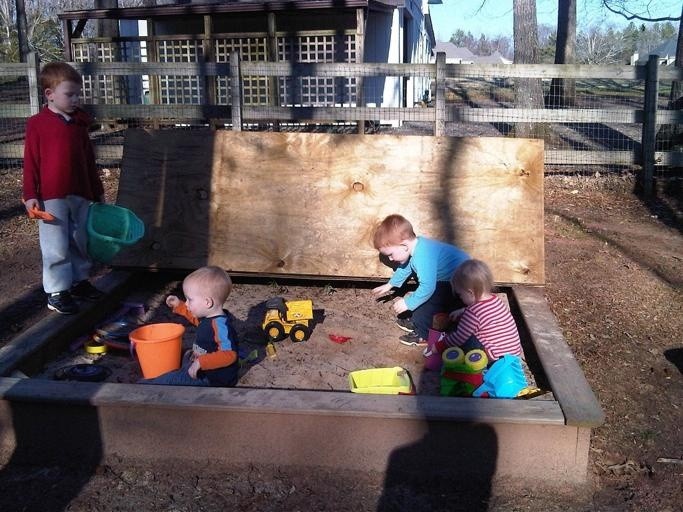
[396,319,416,332]
[46,290,78,315]
[399,330,426,345]
[71,281,107,303]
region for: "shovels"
[21,198,55,223]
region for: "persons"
[134,265,240,387]
[435,257,524,365]
[21,61,106,314]
[372,213,473,346]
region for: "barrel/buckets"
[88,202,145,264]
[129,323,184,380]
